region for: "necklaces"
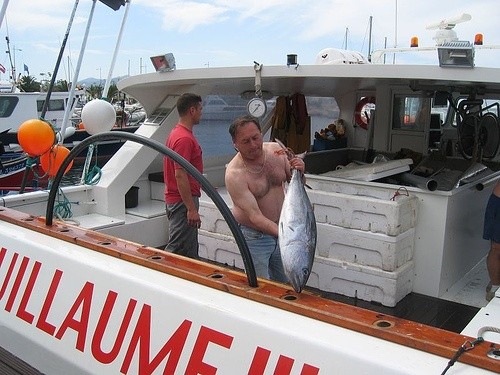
[241,151,266,173]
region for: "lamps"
[436,40,476,69]
[150,53,176,72]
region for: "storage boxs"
[198,179,418,307]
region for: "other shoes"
[486,281,492,292]
[486,290,495,301]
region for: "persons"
[482,180,500,301]
[163,93,203,257]
[225,116,306,284]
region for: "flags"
[0,64,6,74]
[24,64,29,75]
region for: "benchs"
[62,199,168,247]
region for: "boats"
[0,1,500,375]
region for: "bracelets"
[290,169,304,179]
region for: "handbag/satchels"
[313,132,348,151]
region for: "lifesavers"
[354,95,376,131]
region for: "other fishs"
[274,138,317,293]
